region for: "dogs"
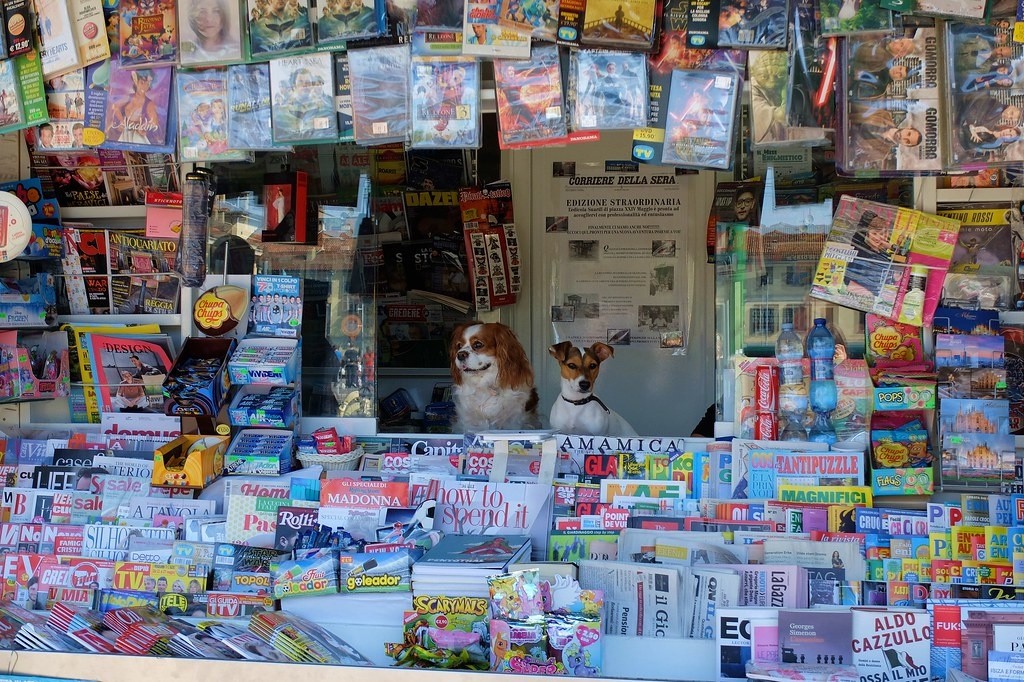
[547,341,639,438]
[447,320,543,434]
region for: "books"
[0,0,1024,682]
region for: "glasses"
[736,198,753,207]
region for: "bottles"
[774,323,807,416]
[809,406,838,450]
[806,318,838,412]
[780,414,807,442]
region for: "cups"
[831,442,867,456]
